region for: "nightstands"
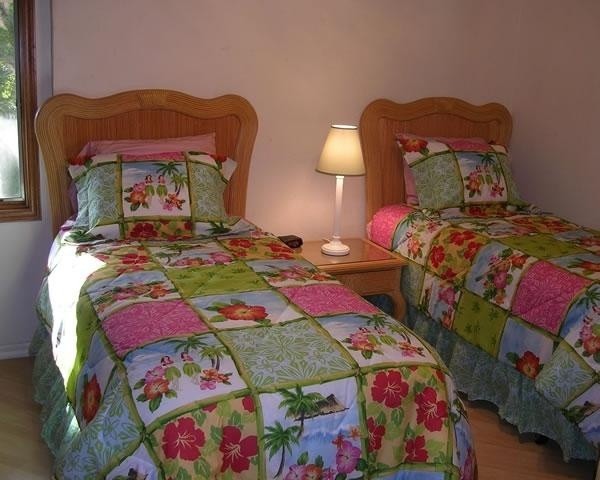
[295,238,408,322]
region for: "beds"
[360,96,600,472]
[29,89,478,479]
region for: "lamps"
[316,125,367,256]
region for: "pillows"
[394,133,528,220]
[401,133,490,206]
[77,133,217,158]
[62,153,256,245]
